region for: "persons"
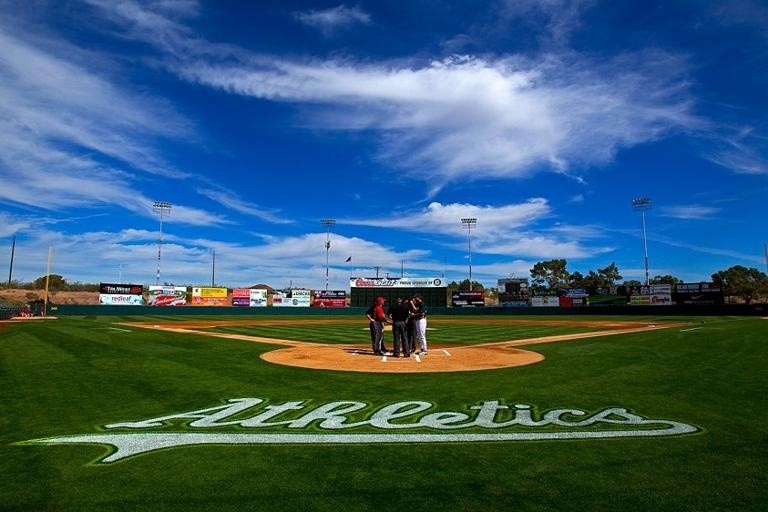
[373,296,390,355]
[390,292,428,357]
[366,300,388,353]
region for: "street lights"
[461,218,477,292]
[320,218,337,291]
[153,201,172,286]
[632,196,652,286]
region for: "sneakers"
[373,350,411,358]
[415,348,428,356]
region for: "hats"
[376,296,387,304]
